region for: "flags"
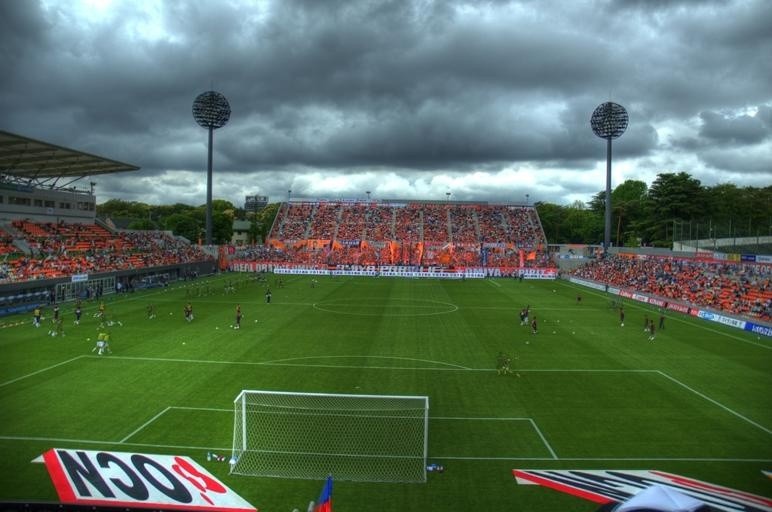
[317,474,333,512]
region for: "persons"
[647,319,657,343]
[644,314,650,331]
[0,216,217,356]
[659,314,666,330]
[494,350,522,379]
[234,304,240,330]
[567,249,772,322]
[265,288,272,305]
[519,303,537,335]
[223,199,560,296]
